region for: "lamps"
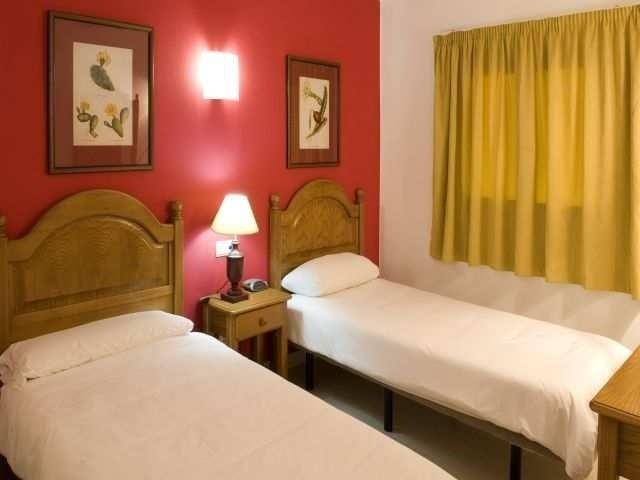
[198,50,243,102]
[208,189,257,301]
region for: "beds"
[1,188,454,480]
[260,178,639,480]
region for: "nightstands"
[200,289,290,381]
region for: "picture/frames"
[45,10,157,172]
[285,55,341,167]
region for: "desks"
[591,334,640,480]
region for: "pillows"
[281,250,383,299]
[0,309,194,387]
